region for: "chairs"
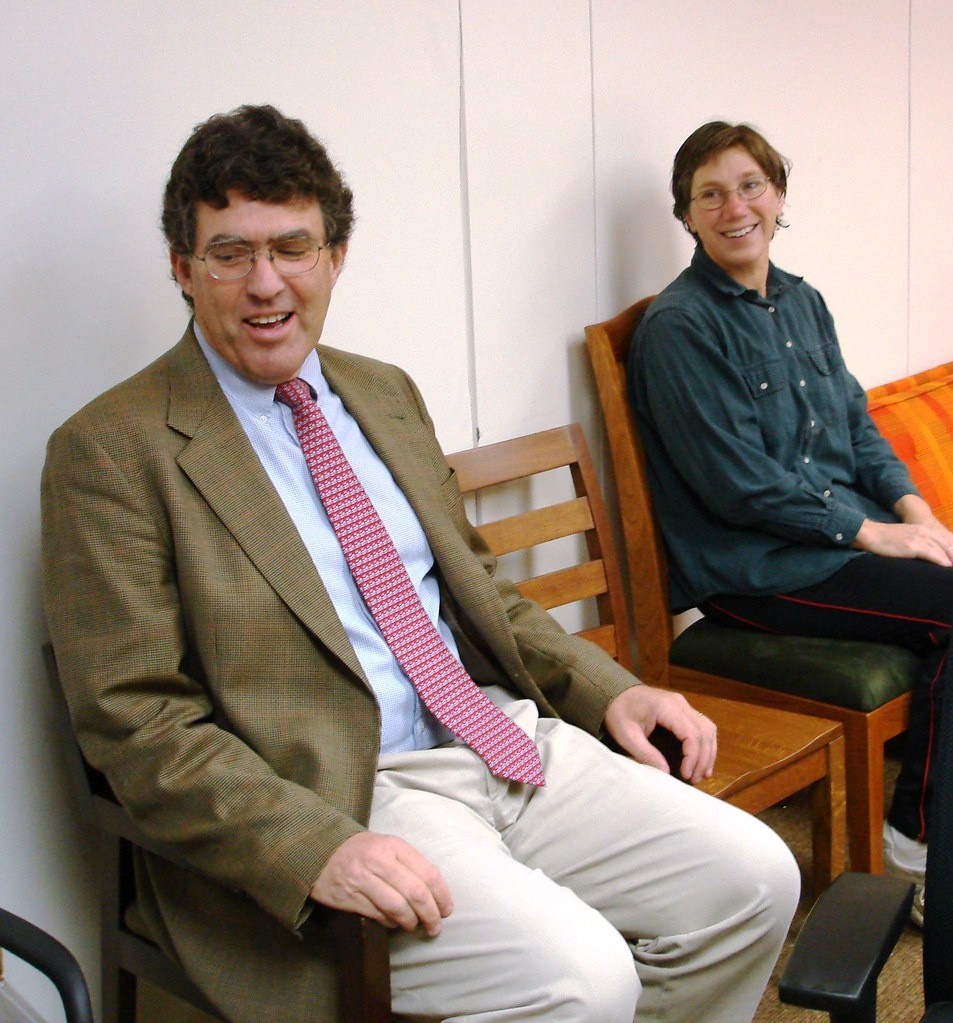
[442,423,849,901]
[41,627,698,1023]
[582,296,915,878]
[778,871,953,1023]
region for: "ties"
[275,378,549,790]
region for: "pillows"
[866,360,953,535]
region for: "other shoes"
[881,819,924,924]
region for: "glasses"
[688,175,770,211]
[192,239,333,281]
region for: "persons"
[627,121,952,925]
[40,104,803,1019]
